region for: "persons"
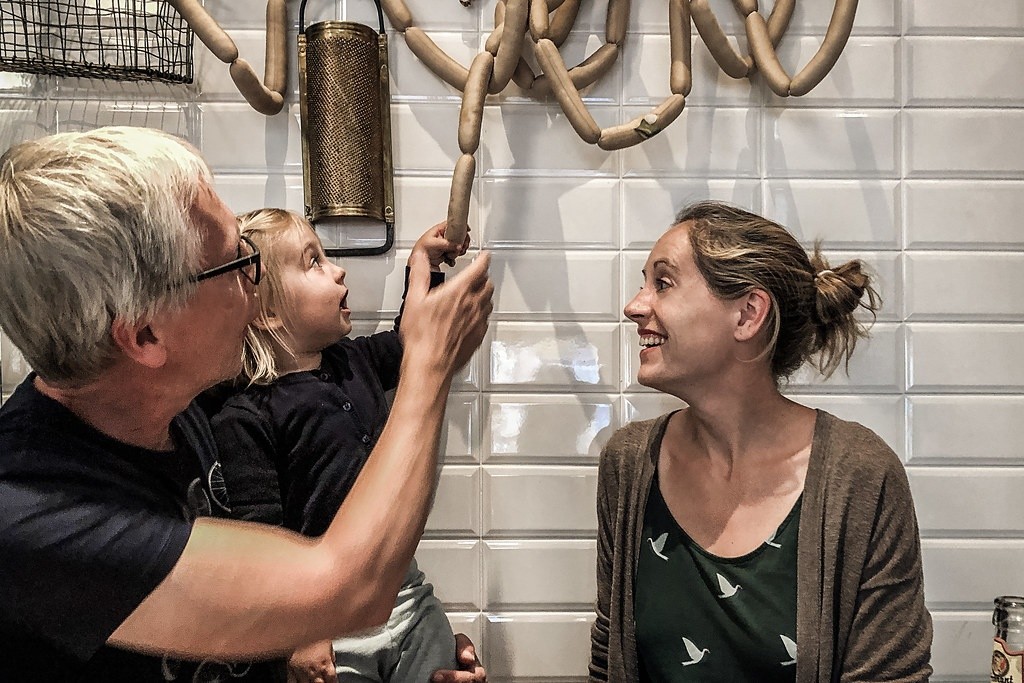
[0,126,496,683]
[209,208,471,683]
[587,203,933,683]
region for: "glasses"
[167,234,261,290]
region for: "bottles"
[989,595,1024,683]
